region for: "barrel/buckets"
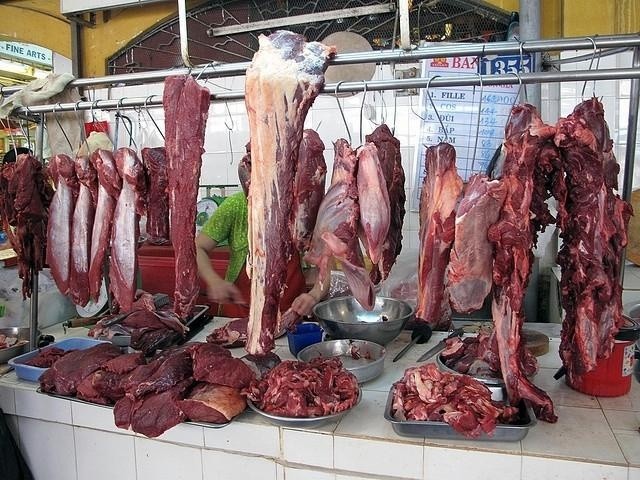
[563,311,640,395]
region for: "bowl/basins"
[312,294,415,344]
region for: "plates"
[297,339,387,384]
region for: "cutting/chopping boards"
[479,326,550,355]
[625,187,639,266]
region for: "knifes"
[418,327,464,362]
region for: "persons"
[195,191,337,322]
